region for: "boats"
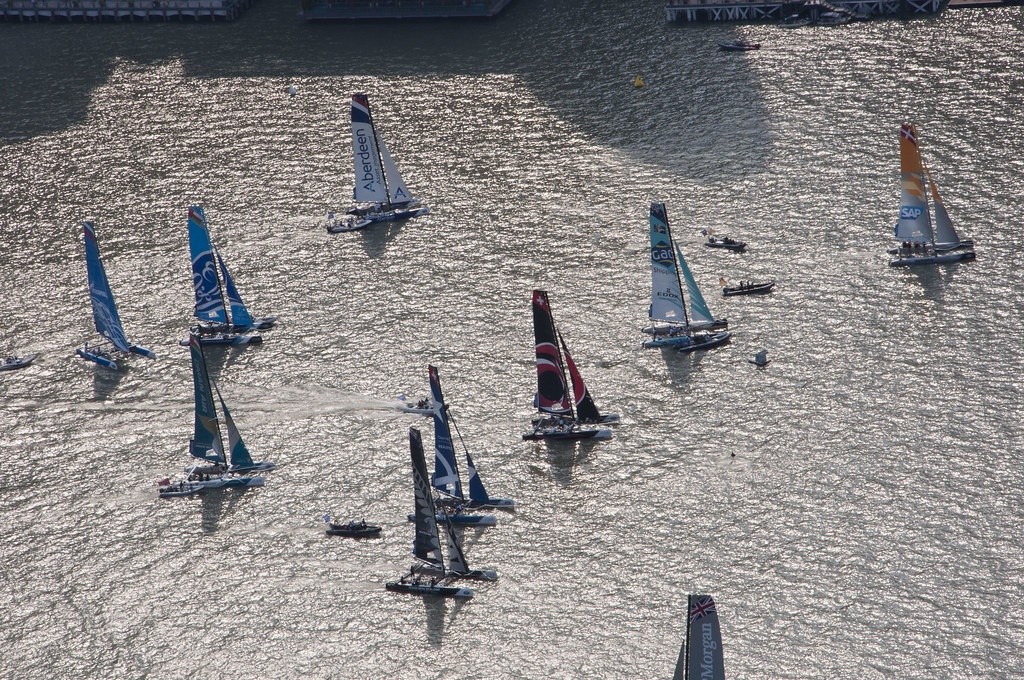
[326,517,382,535]
[718,276,774,295]
[701,229,747,250]
[0,353,38,370]
[718,40,761,51]
[404,396,450,416]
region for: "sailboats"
[180,205,279,346]
[641,200,732,353]
[888,121,976,267]
[325,92,429,233]
[522,289,620,440]
[385,425,498,598]
[407,364,514,526]
[74,219,158,371]
[158,326,275,498]
